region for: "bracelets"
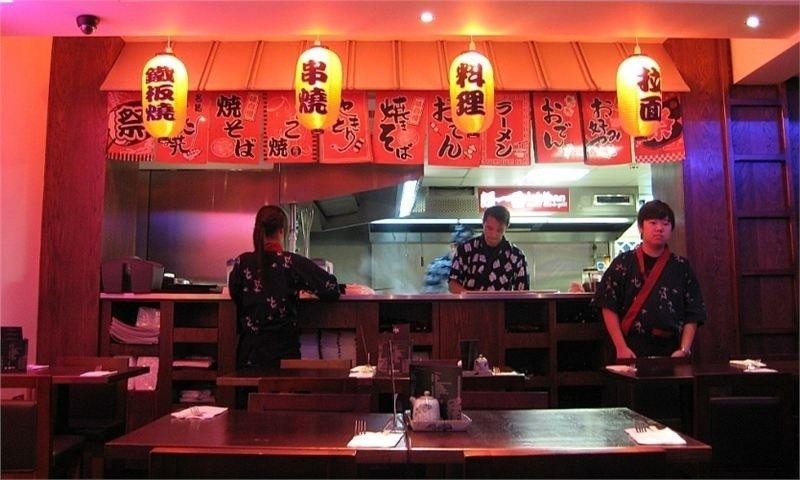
[681,345,692,354]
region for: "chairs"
[459,445,664,477]
[146,445,360,478]
[0,372,85,478]
[665,369,796,477]
[48,357,130,441]
[205,354,554,409]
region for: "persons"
[593,200,707,359]
[446,206,531,293]
[423,227,473,288]
[228,206,341,408]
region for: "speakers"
[101,260,127,292]
[131,260,164,293]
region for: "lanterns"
[295,47,343,131]
[140,51,188,138]
[616,54,662,140]
[449,50,495,139]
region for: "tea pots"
[472,353,491,375]
[409,390,442,421]
